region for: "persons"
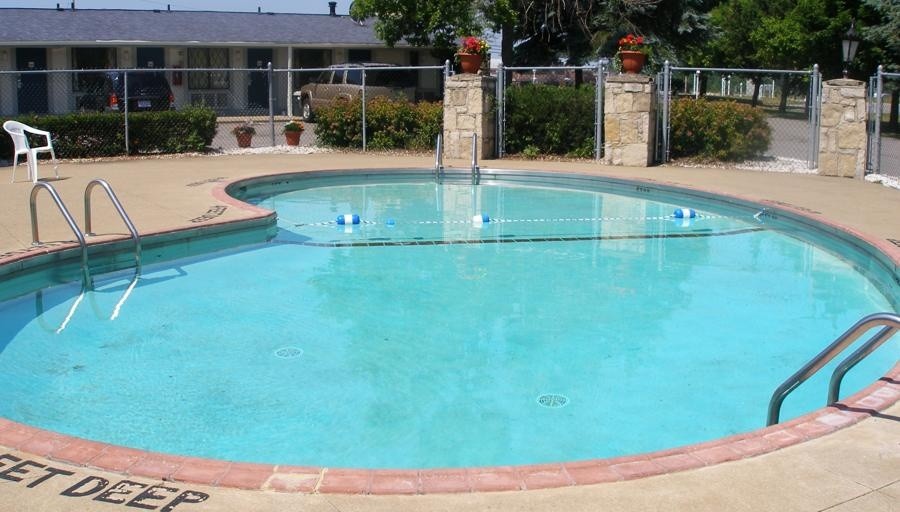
[21,59,46,88]
[250,60,267,86]
[147,60,155,68]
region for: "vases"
[620,51,645,74]
[457,53,482,73]
[284,131,301,145]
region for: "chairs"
[2,120,61,186]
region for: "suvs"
[75,70,175,111]
[297,62,416,121]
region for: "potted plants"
[230,126,255,148]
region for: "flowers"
[454,37,491,66]
[614,33,656,74]
[280,118,304,136]
